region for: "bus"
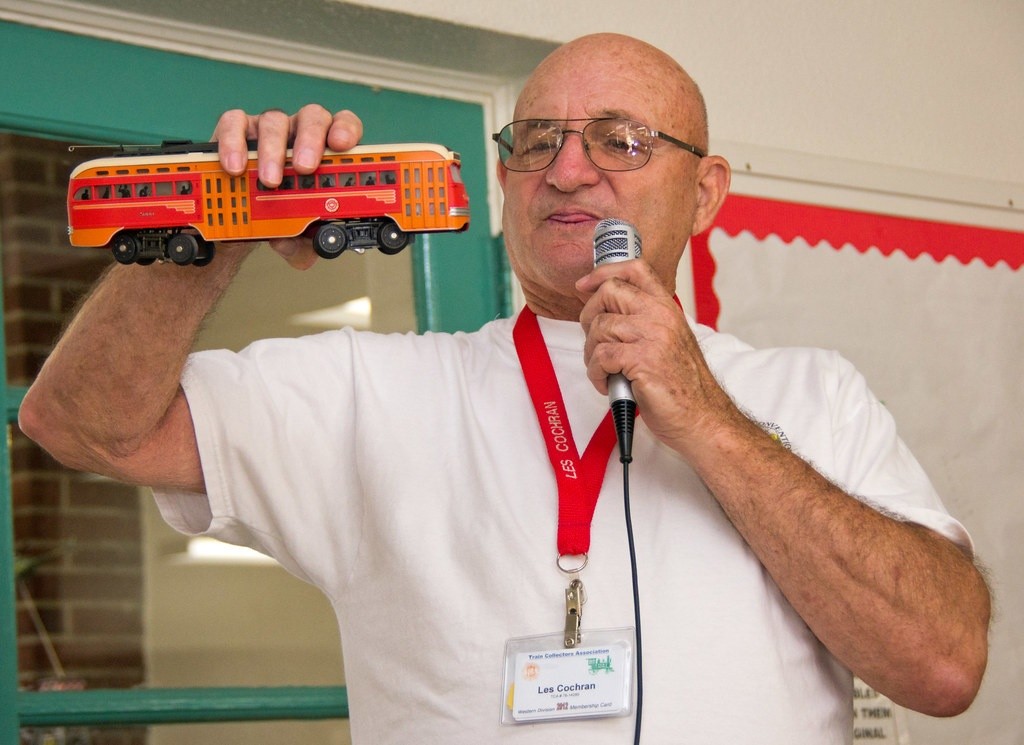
[66,135,474,266]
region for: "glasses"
[493,116,703,172]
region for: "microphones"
[594,218,643,459]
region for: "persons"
[17,31,990,745]
